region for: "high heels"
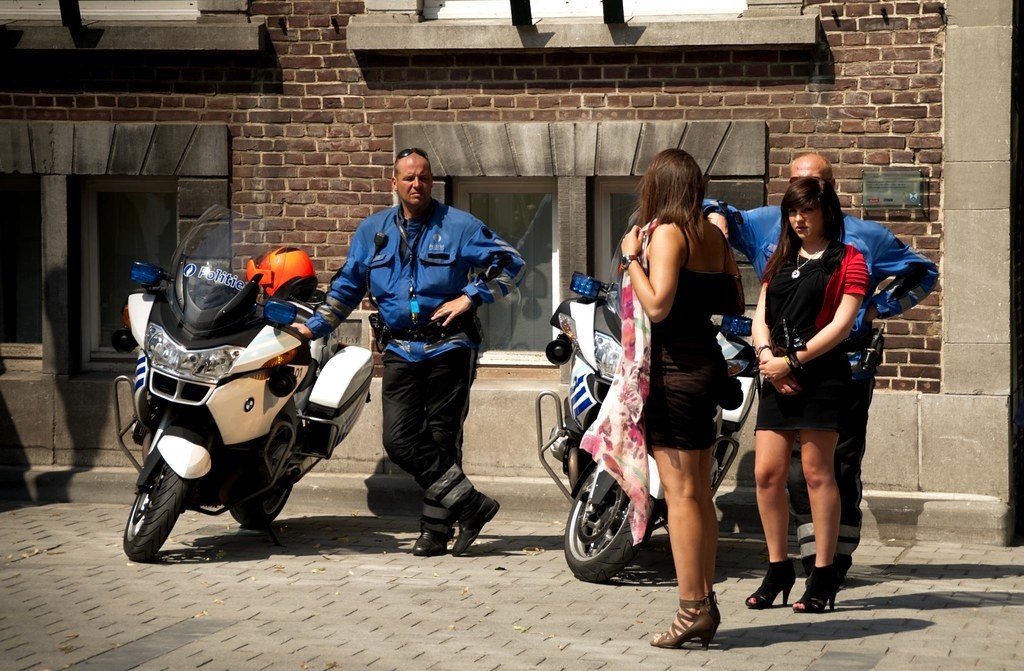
[650,600,712,650]
[793,562,841,613]
[705,591,720,644]
[745,557,796,608]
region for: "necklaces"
[791,244,825,279]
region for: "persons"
[702,154,938,579]
[746,176,869,615]
[291,149,528,555]
[621,147,746,651]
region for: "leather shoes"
[413,529,447,556]
[453,492,500,556]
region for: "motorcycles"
[536,273,764,583]
[110,203,376,564]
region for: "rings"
[765,376,768,379]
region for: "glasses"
[395,148,429,160]
[789,177,819,184]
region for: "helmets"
[246,245,319,300]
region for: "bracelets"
[784,351,802,372]
[757,344,770,356]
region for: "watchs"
[621,255,638,270]
